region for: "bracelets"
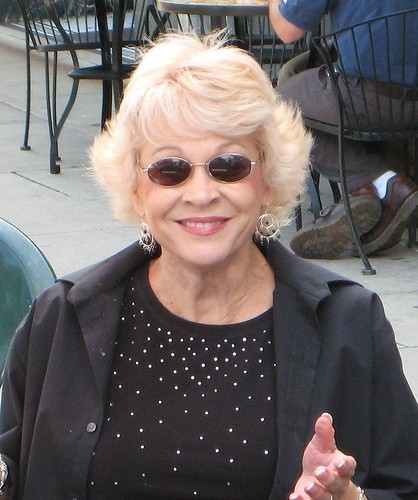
[356,486,367,500]
[0,460,8,496]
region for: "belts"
[343,75,418,101]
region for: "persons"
[0,31,418,500]
[270,0,416,262]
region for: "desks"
[155,0,288,51]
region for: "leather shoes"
[289,182,383,259]
[349,172,418,257]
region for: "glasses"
[139,146,265,188]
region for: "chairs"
[0,217,56,388]
[15,0,173,174]
[297,6,417,276]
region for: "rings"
[329,492,333,500]
